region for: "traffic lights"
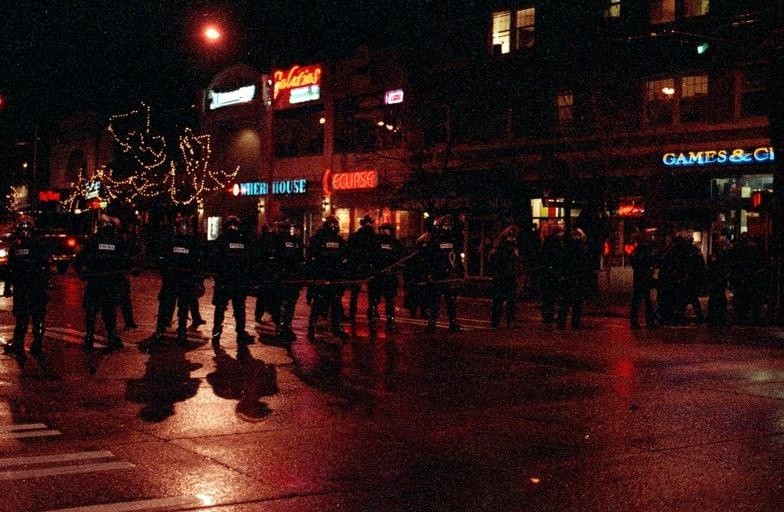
[749,190,768,207]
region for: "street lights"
[17,120,41,215]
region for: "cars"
[0,229,74,276]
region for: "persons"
[630,226,782,330]
[488,223,603,329]
[4,216,139,359]
[151,214,465,347]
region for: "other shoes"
[212,326,223,336]
[236,329,254,344]
[254,310,349,344]
[350,309,461,332]
[149,310,206,345]
[82,308,138,346]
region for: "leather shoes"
[5,339,25,353]
[29,340,43,355]
[488,306,579,326]
[631,313,731,332]
